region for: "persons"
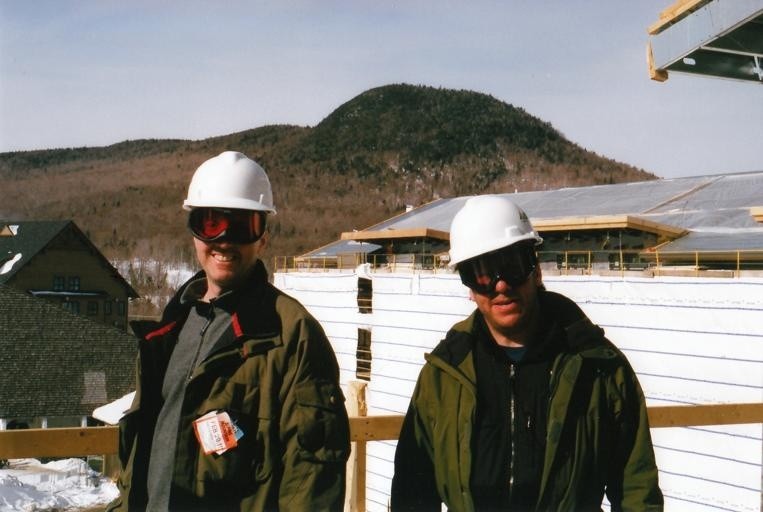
[389,194,665,512]
[104,152,350,512]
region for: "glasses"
[456,241,537,296]
[188,208,269,245]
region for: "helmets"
[447,193,544,271]
[182,150,277,216]
